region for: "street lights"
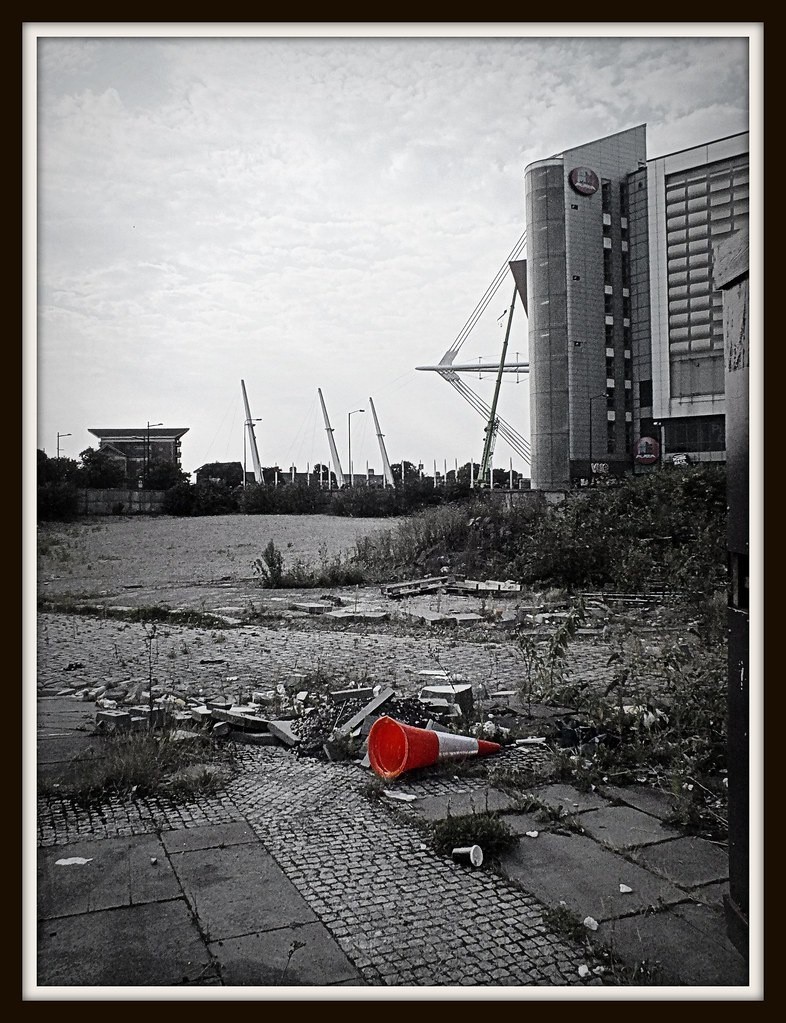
[243,418,263,491]
[348,409,366,487]
[57,432,72,462]
[588,392,609,487]
[146,423,163,483]
[132,435,148,486]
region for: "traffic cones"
[366,716,501,781]
